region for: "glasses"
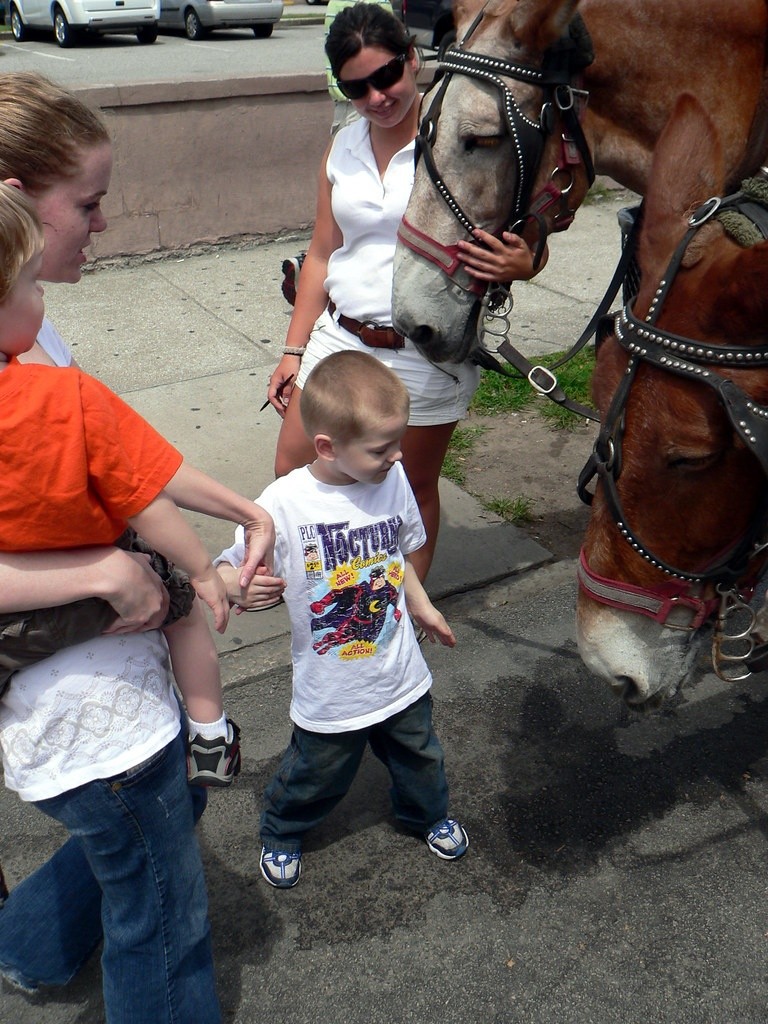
[336,52,405,100]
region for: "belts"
[328,301,405,349]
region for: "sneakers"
[423,821,471,861]
[185,717,241,786]
[259,841,301,887]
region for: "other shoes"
[0,969,39,994]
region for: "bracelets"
[281,346,305,355]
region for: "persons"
[267,0,549,644]
[0,70,276,1024]
[213,350,469,889]
[0,186,241,784]
[282,0,393,308]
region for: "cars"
[8,0,160,48]
[157,0,283,42]
[392,0,455,49]
